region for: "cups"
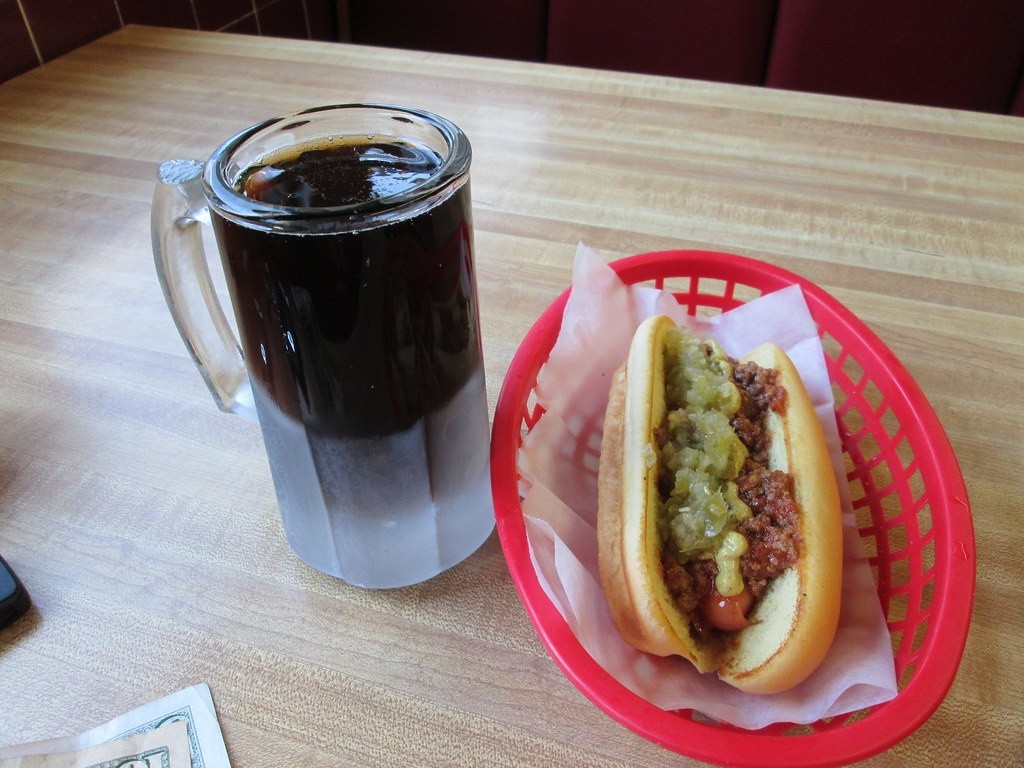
[149,101,495,590]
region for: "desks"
[0,23,1024,768]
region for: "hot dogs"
[595,314,842,695]
